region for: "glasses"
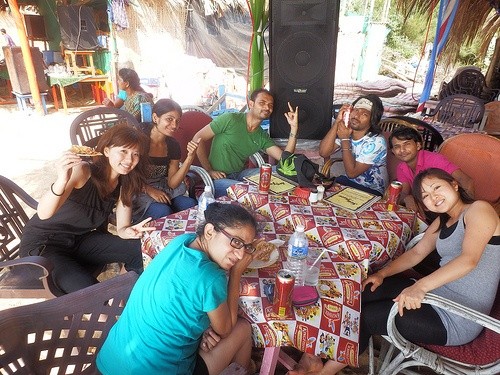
[213,222,256,254]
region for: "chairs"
[70,107,139,145]
[0,175,82,354]
[173,111,265,200]
[379,70,500,375]
[0,271,139,375]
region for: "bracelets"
[51,183,65,196]
[341,149,352,150]
[201,160,209,167]
[290,132,299,138]
[340,138,351,141]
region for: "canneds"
[342,105,353,128]
[386,181,403,211]
[259,164,272,193]
[272,268,295,316]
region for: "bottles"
[309,189,318,202]
[317,186,325,201]
[195,186,214,231]
[286,225,309,287]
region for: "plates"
[246,248,279,269]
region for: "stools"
[11,91,47,118]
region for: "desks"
[64,50,96,76]
[46,73,109,115]
[140,182,428,375]
[248,138,322,169]
[424,100,488,131]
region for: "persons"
[133,98,201,223]
[95,202,258,375]
[319,95,389,199]
[389,127,475,237]
[103,68,156,128]
[0,28,18,48]
[192,89,298,198]
[19,125,144,297]
[285,168,500,375]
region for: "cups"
[304,250,322,286]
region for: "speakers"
[25,14,46,38]
[269,0,340,140]
[3,45,48,95]
[58,5,97,53]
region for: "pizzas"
[71,145,98,155]
[254,242,275,262]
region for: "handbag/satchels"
[276,151,337,188]
